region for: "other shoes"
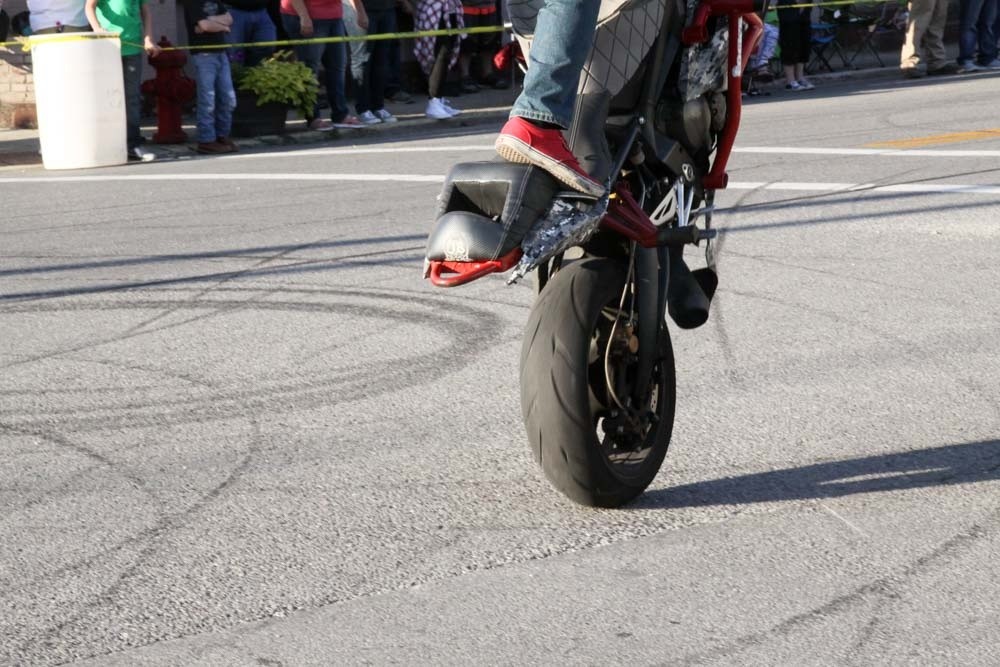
[197,142,232,153]
[926,64,966,74]
[956,60,979,73]
[901,68,921,78]
[978,58,1000,70]
[129,146,156,161]
[217,136,239,152]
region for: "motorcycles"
[422,0,765,511]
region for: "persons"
[185,0,238,154]
[84,0,162,162]
[752,23,779,82]
[494,0,608,198]
[957,0,1000,73]
[900,0,958,78]
[224,0,467,131]
[778,0,815,91]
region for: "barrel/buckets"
[30,33,130,170]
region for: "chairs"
[805,14,884,75]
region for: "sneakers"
[425,97,461,120]
[375,109,398,123]
[308,118,334,131]
[785,80,806,91]
[333,114,368,128]
[495,116,606,198]
[383,92,415,104]
[359,110,381,124]
[798,80,815,89]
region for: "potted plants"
[235,53,320,137]
